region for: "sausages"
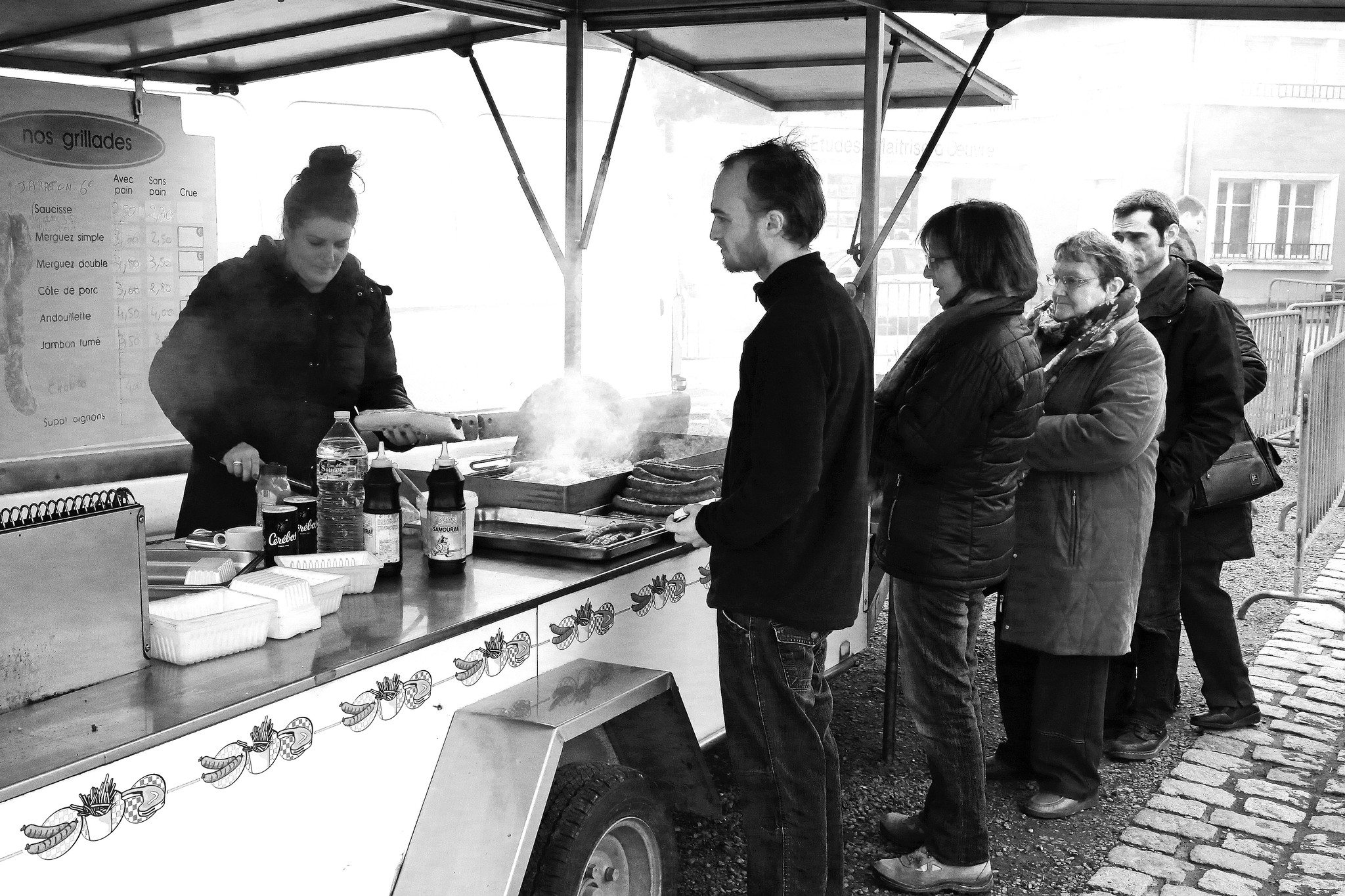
[0,210,37,416]
[611,460,724,523]
[550,521,656,545]
[496,457,634,486]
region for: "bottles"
[426,441,466,577]
[255,461,291,527]
[316,411,367,553]
[362,441,402,579]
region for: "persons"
[872,199,1047,894]
[1105,190,1267,762]
[147,145,430,541]
[663,133,875,896]
[983,227,1169,818]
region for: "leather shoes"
[1111,726,1169,761]
[1191,700,1259,728]
[1023,791,1100,818]
[984,755,1014,774]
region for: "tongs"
[255,468,312,495]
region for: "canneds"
[261,505,299,569]
[282,496,318,554]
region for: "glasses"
[925,255,952,270]
[1046,273,1106,288]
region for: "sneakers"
[872,844,993,893]
[881,812,922,845]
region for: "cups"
[213,526,263,551]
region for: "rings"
[233,461,242,466]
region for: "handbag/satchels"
[1190,436,1283,509]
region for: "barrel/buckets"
[415,489,478,557]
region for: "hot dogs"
[353,410,466,441]
